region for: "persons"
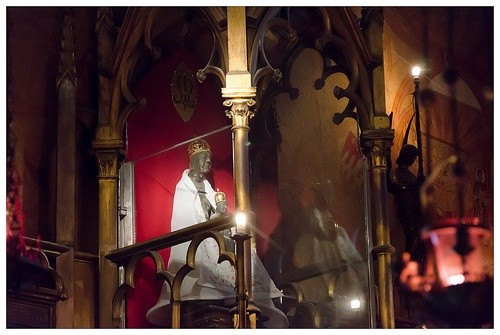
[206,186,236,256]
[157,138,284,307]
[387,143,429,262]
